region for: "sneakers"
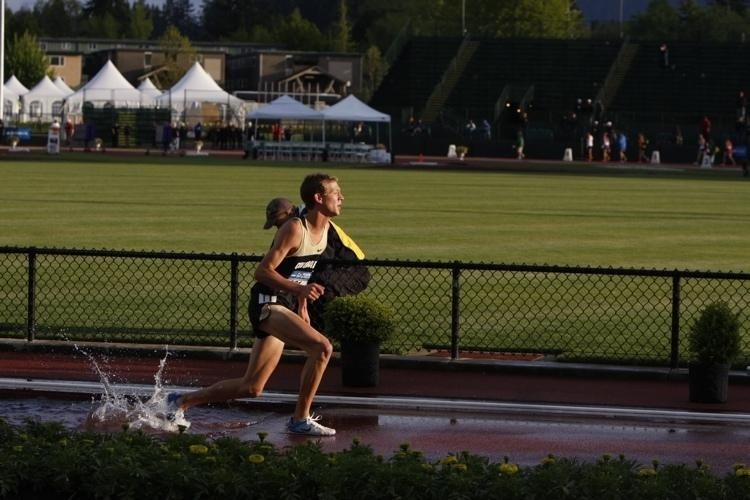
[288,412,336,436]
[167,393,189,427]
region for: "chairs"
[245,140,384,163]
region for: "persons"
[244,125,292,149]
[582,122,628,161]
[406,115,428,135]
[110,121,137,147]
[736,90,746,131]
[693,127,736,168]
[64,117,73,151]
[636,130,650,163]
[51,119,61,128]
[462,118,491,145]
[163,122,244,155]
[251,197,373,335]
[512,113,527,161]
[156,172,347,441]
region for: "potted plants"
[456,145,469,161]
[685,300,744,404]
[194,140,203,153]
[92,137,103,151]
[324,294,394,388]
[8,135,20,150]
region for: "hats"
[264,198,293,229]
